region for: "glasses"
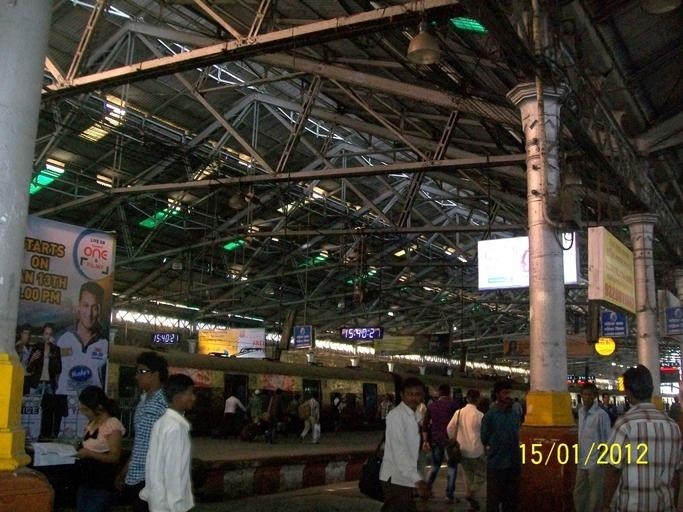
[136,369,150,374]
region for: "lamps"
[404,17,442,68]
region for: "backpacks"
[329,400,341,419]
[298,402,311,420]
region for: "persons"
[599,364,683,511]
[15,323,34,397]
[55,281,109,440]
[115,351,169,511]
[422,384,461,504]
[222,388,355,447]
[511,397,524,421]
[572,382,613,512]
[374,393,395,442]
[481,380,524,512]
[139,373,196,511]
[669,396,681,423]
[70,385,126,512]
[446,389,485,512]
[601,393,618,427]
[26,322,63,441]
[379,377,431,512]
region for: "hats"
[466,388,480,399]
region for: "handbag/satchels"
[445,439,460,467]
[359,454,385,502]
